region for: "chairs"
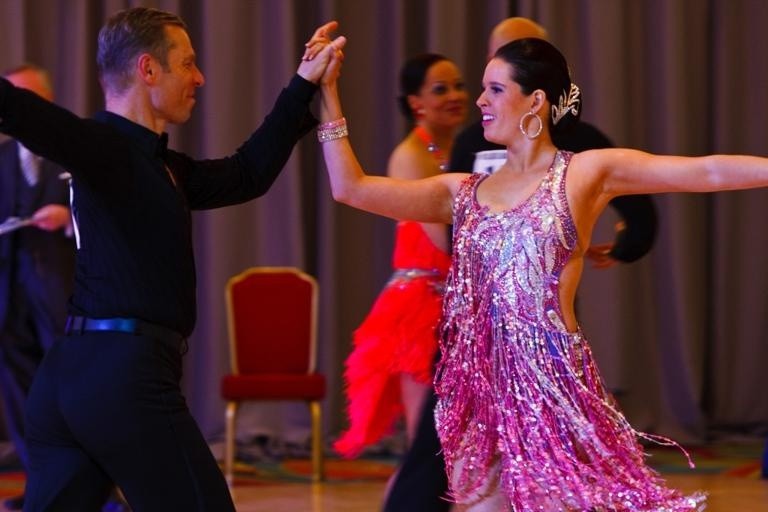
[220,265,328,490]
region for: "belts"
[65,316,189,358]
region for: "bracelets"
[317,116,349,143]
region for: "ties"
[19,152,40,186]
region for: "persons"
[330,51,472,508]
[381,15,662,511]
[0,5,348,512]
[0,65,127,512]
[300,29,768,511]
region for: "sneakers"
[4,493,26,509]
